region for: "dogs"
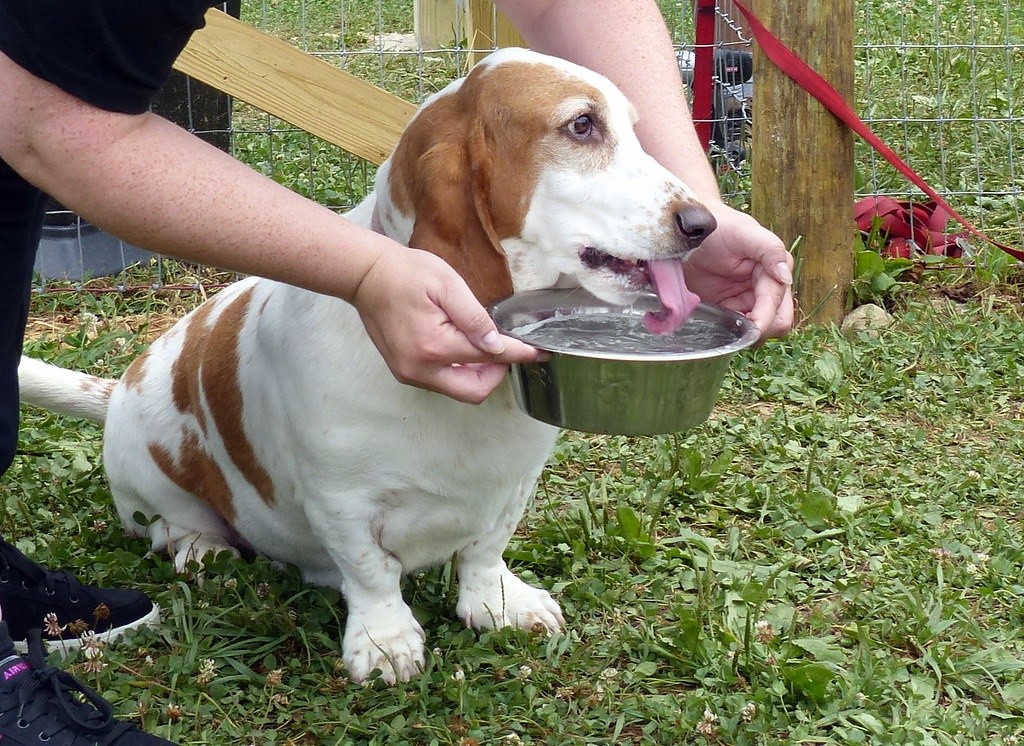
[16,46,717,691]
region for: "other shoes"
[726,107,753,151]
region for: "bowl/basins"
[492,289,760,434]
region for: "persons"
[689,0,754,165]
[0,0,795,746]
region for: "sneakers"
[1,541,160,656]
[1,654,180,746]
